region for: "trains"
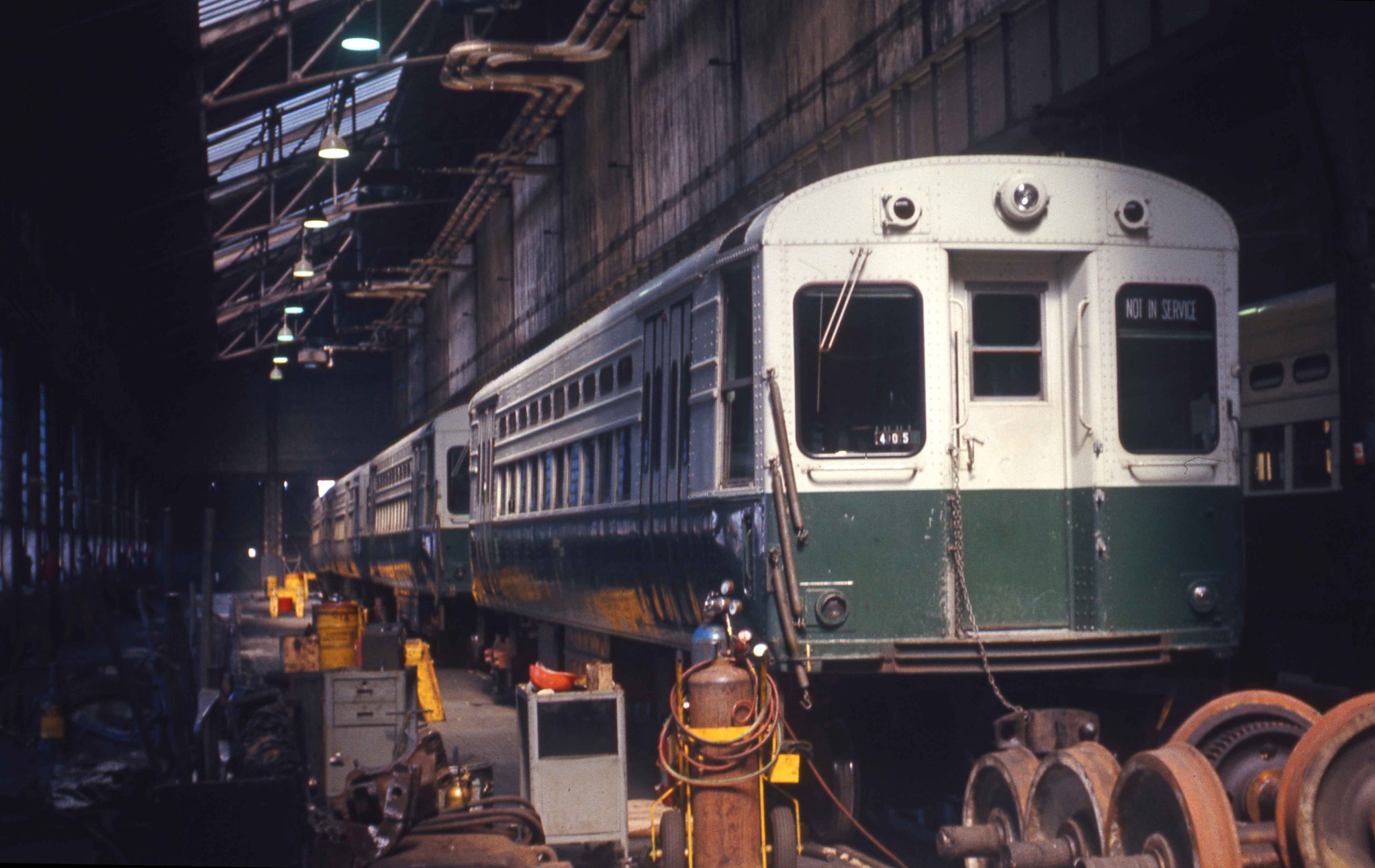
[311,155,1265,846]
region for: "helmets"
[529,664,584,690]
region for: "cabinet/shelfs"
[322,664,411,799]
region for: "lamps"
[339,15,381,52]
[318,82,350,160]
[283,286,304,314]
[292,228,315,277]
[302,194,329,229]
[269,362,283,380]
[276,301,294,341]
